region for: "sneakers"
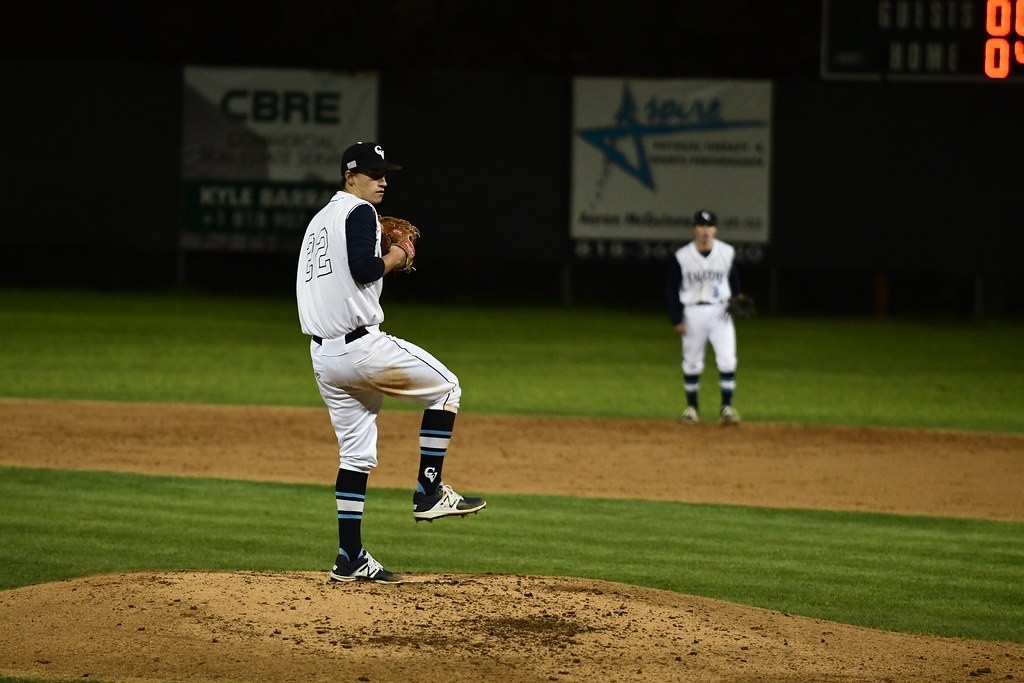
[719,405,739,423]
[680,406,700,424]
[411,485,487,523]
[329,551,406,584]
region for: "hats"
[692,210,719,226]
[341,141,402,180]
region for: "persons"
[297,141,486,583]
[665,209,741,425]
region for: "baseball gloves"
[378,214,420,271]
